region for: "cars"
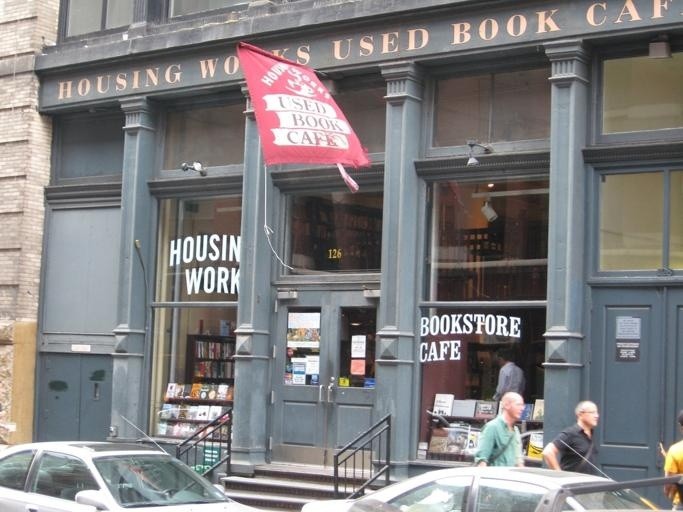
[0,441,268,510]
[303,466,680,511]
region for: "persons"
[663,411,683,511]
[492,345,527,403]
[543,400,601,473]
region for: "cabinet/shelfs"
[184,334,235,383]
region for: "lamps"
[481,198,498,223]
[466,139,493,167]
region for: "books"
[427,392,545,467]
[157,341,233,440]
